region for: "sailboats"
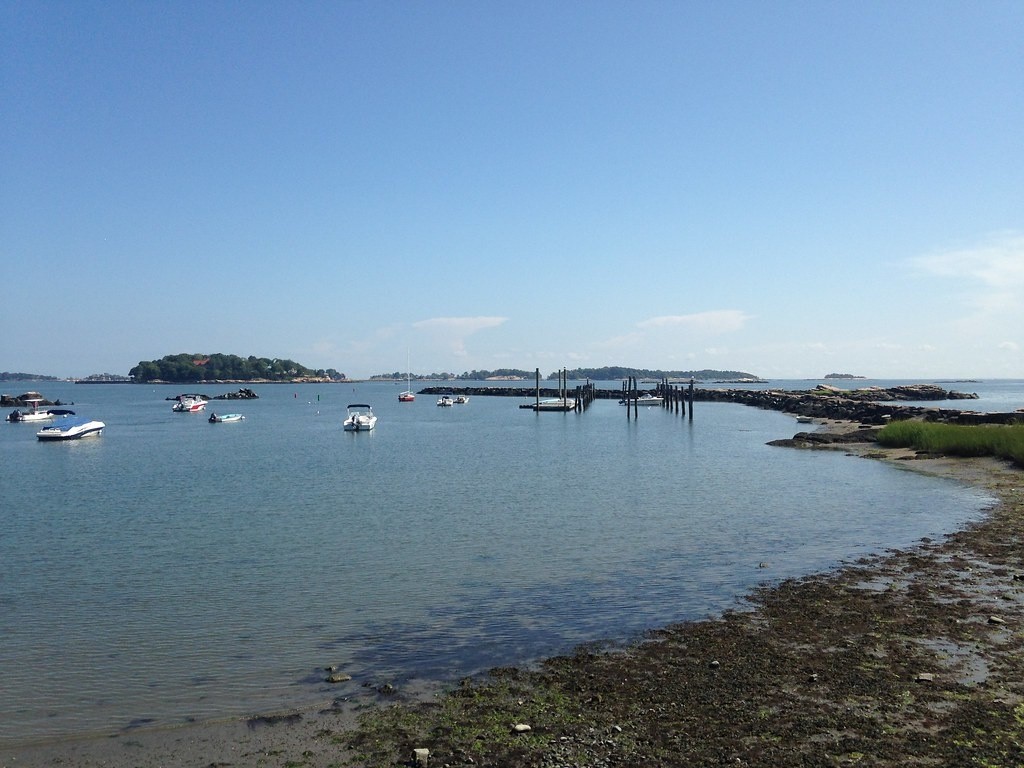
[396,351,415,401]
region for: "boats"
[343,403,377,431]
[172,395,208,413]
[436,394,470,406]
[36,419,106,442]
[5,398,55,423]
[795,414,814,423]
[208,412,243,424]
[626,394,663,407]
